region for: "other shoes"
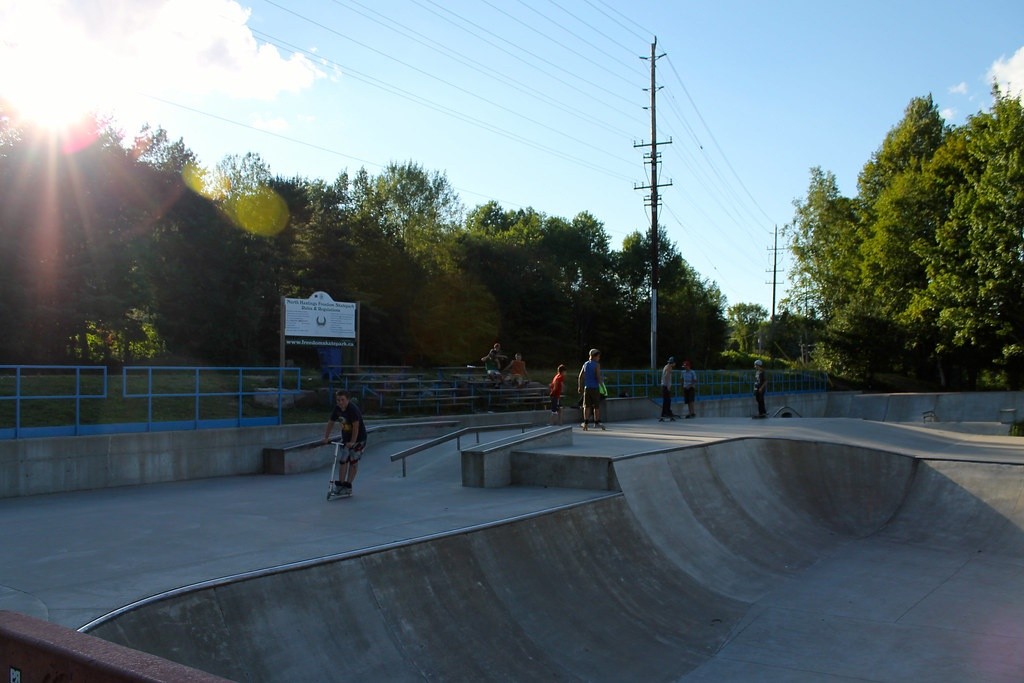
[686,413,694,418]
[340,483,353,495]
[581,421,589,428]
[333,481,344,493]
[594,421,604,428]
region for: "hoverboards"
[323,439,356,501]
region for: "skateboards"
[656,416,679,422]
[752,413,770,419]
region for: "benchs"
[326,365,565,417]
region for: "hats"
[668,356,676,363]
[589,348,598,360]
[755,360,762,367]
[682,361,690,367]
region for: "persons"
[480,343,508,388]
[503,353,529,387]
[680,360,697,417]
[661,356,676,417]
[550,364,568,414]
[580,379,607,407]
[578,348,602,428]
[752,359,768,415]
[323,389,367,494]
